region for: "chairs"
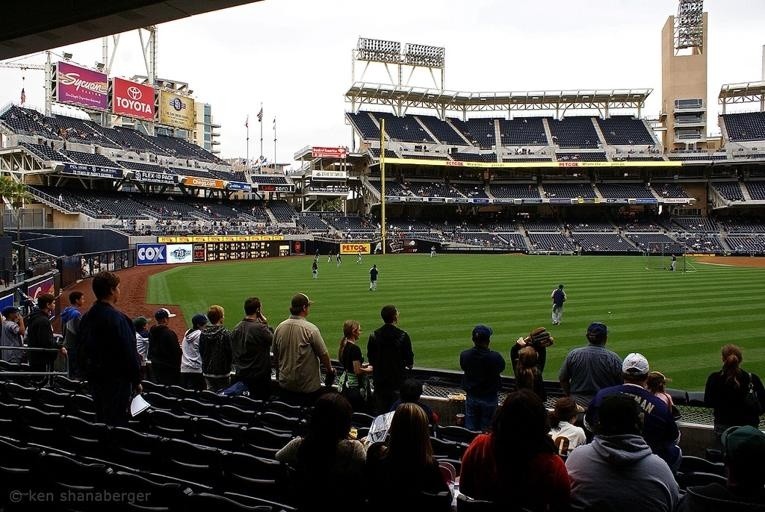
[257,411,296,431]
[675,470,727,491]
[162,437,221,479]
[242,427,289,453]
[44,453,107,512]
[182,399,216,416]
[62,415,108,457]
[22,406,64,447]
[6,383,40,406]
[232,393,264,410]
[194,418,239,450]
[429,435,468,459]
[148,392,181,411]
[55,376,87,392]
[109,426,163,472]
[437,422,482,442]
[0,437,40,509]
[149,411,192,441]
[0,402,20,436]
[679,455,726,475]
[183,489,282,512]
[167,384,196,399]
[111,472,179,511]
[216,405,255,424]
[40,388,71,410]
[199,390,226,404]
[221,450,284,488]
[140,378,163,390]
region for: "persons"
[565,392,679,512]
[356,253,362,264]
[61,291,85,351]
[546,397,586,458]
[198,305,233,390]
[336,251,342,263]
[670,253,677,272]
[312,259,319,279]
[678,425,765,512]
[644,370,675,409]
[430,245,437,258]
[133,316,149,368]
[456,388,570,512]
[550,284,567,326]
[27,294,67,372]
[314,249,321,263]
[558,322,624,408]
[180,314,210,384]
[1,306,25,364]
[367,304,414,405]
[58,192,63,206]
[217,226,282,235]
[344,228,376,240]
[363,402,453,512]
[703,343,765,436]
[509,237,514,248]
[369,264,379,293]
[270,293,338,398]
[339,320,374,383]
[459,325,505,429]
[389,377,432,421]
[228,297,274,391]
[276,393,366,507]
[327,249,332,262]
[75,271,144,425]
[510,327,554,404]
[583,352,668,443]
[149,308,183,382]
[440,235,494,245]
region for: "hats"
[135,317,153,325]
[587,322,609,335]
[567,399,584,413]
[155,307,176,319]
[472,325,493,338]
[190,314,208,322]
[724,425,765,460]
[622,351,650,376]
